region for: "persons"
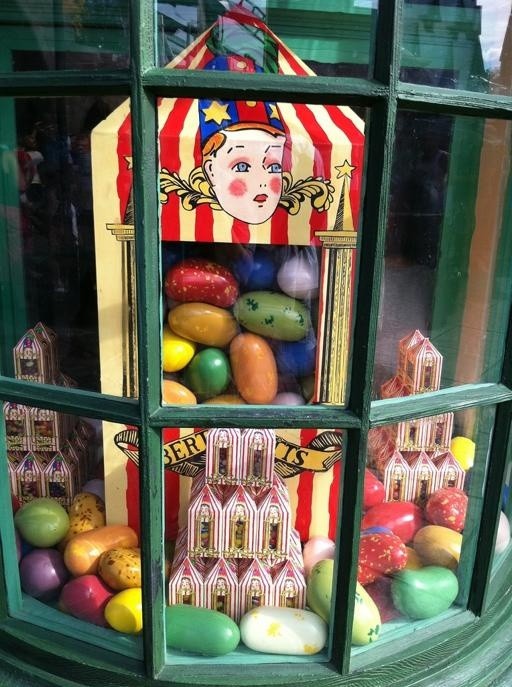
[199,58,287,224]
